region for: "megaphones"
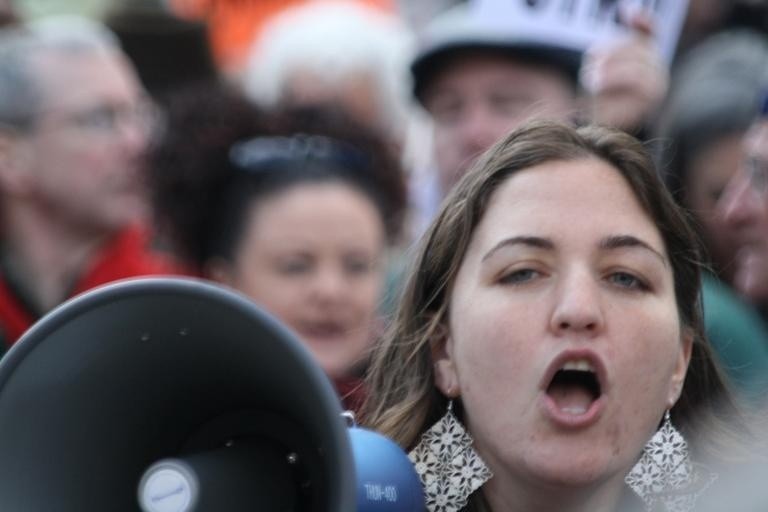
[0,276,426,512]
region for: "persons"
[1,1,768,439]
[368,111,768,512]
